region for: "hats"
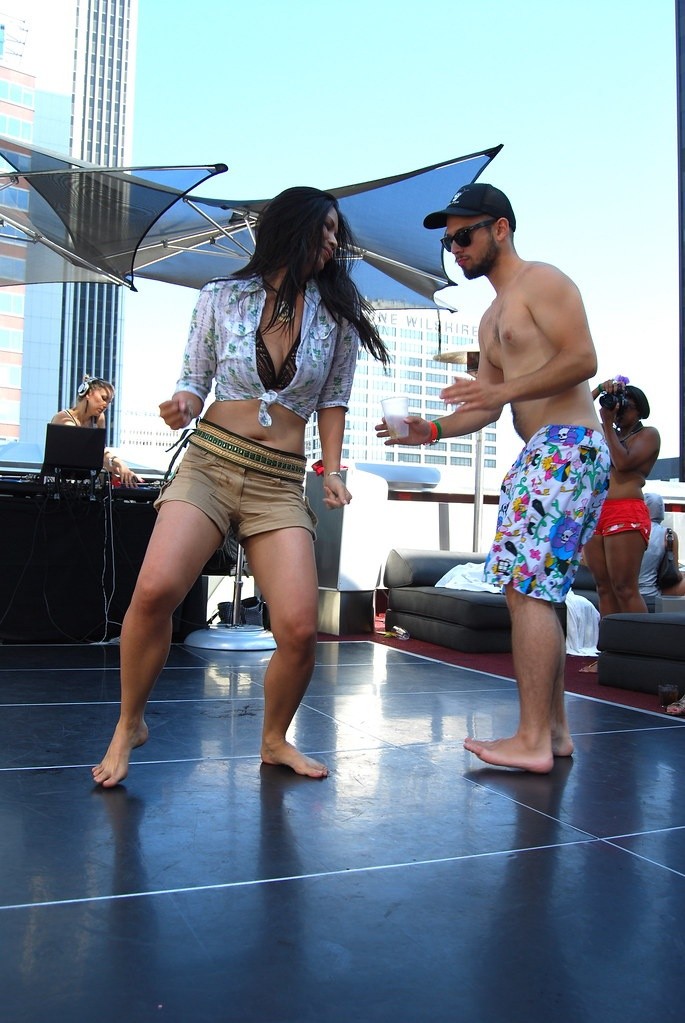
[624,385,651,420]
[422,184,516,233]
[643,494,666,522]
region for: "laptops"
[36,425,108,485]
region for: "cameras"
[599,389,628,412]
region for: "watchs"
[108,455,118,468]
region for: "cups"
[390,625,409,641]
[380,396,410,439]
[659,683,679,708]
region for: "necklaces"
[273,299,295,323]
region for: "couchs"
[383,548,685,696]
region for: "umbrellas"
[1,164,230,292]
[1,136,506,626]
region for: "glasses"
[440,219,498,252]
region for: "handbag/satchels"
[206,595,264,630]
[656,529,682,589]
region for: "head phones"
[77,377,104,398]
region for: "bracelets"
[326,471,344,480]
[597,384,603,393]
[428,419,442,445]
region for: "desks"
[0,495,208,644]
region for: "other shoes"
[665,696,685,716]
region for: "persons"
[579,376,660,672]
[51,374,147,488]
[642,492,685,613]
[90,186,397,786]
[375,182,614,773]
